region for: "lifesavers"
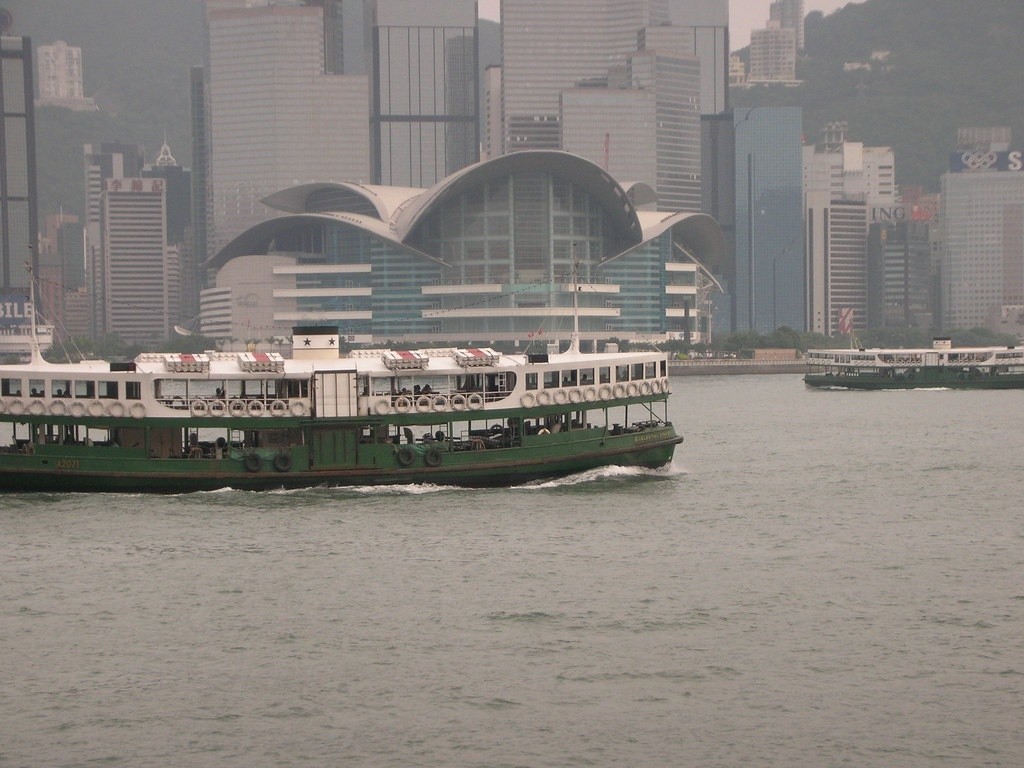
[0,397,6,413]
[399,446,443,467]
[243,453,293,472]
[88,400,105,417]
[854,361,877,367]
[28,398,47,416]
[69,402,86,418]
[228,400,306,418]
[538,428,550,435]
[210,400,227,417]
[994,359,1019,364]
[48,400,66,416]
[889,361,921,366]
[7,399,27,415]
[190,399,208,418]
[108,401,126,418]
[948,359,981,366]
[128,402,148,420]
[806,358,831,365]
[375,377,669,415]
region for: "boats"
[1,260,684,492]
[803,345,1024,389]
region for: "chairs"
[188,433,202,459]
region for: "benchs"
[384,392,440,405]
